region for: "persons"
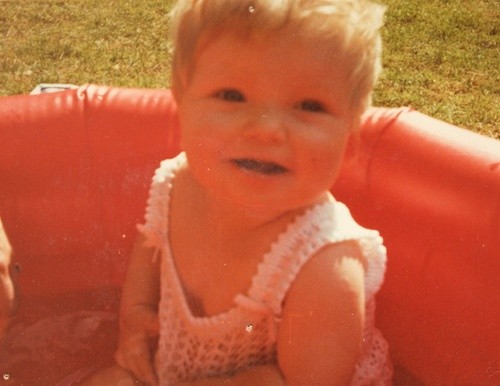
[0,215,18,332]
[68,0,402,386]
